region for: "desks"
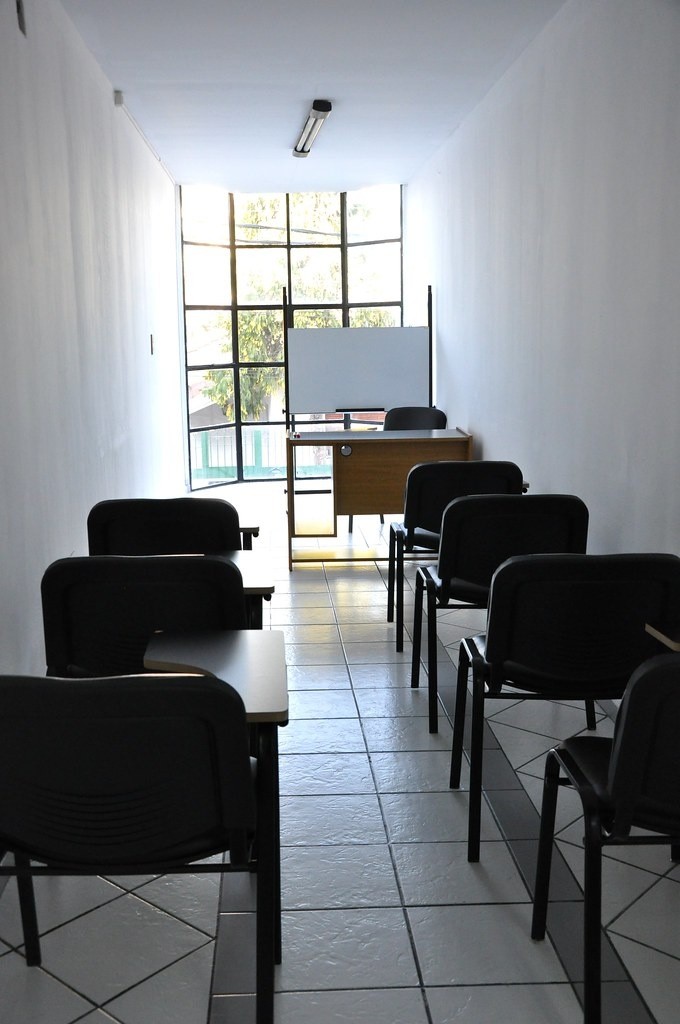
[143,629,288,857]
[211,547,275,629]
[286,427,473,571]
[239,518,260,550]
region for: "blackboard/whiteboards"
[285,326,431,415]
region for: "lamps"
[293,101,332,158]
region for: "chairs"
[350,406,446,534]
[450,553,679,863]
[0,674,282,1024]
[86,498,242,552]
[386,460,523,653]
[40,557,244,679]
[531,653,679,1024]
[410,495,589,735]
